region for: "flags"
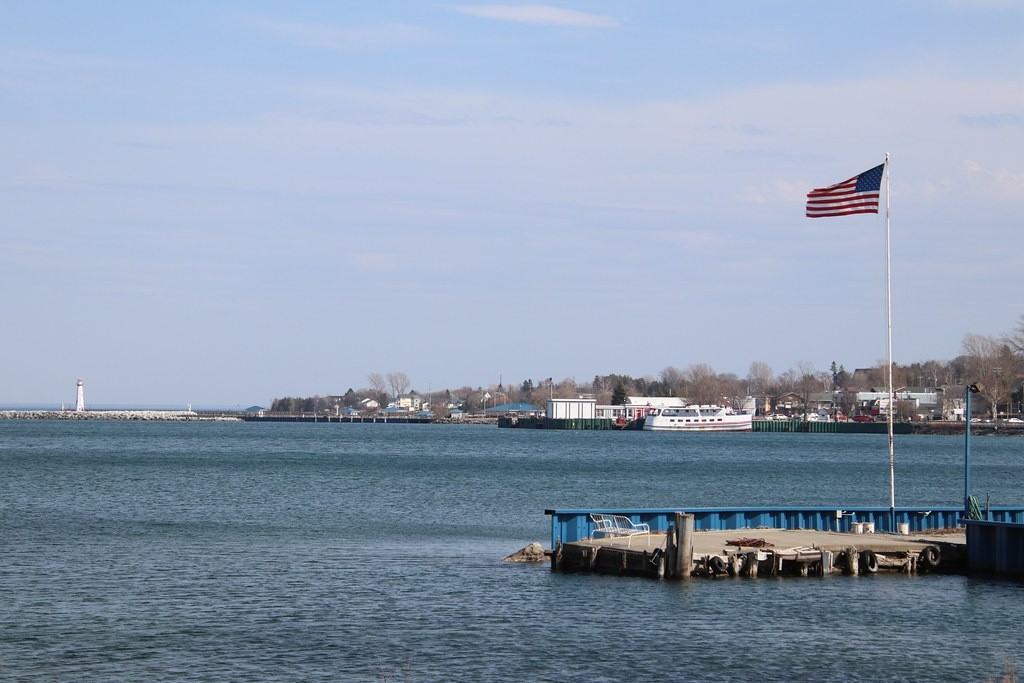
[805,162,886,218]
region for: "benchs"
[590,513,650,548]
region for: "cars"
[764,413,819,421]
[971,418,1023,422]
[831,413,846,421]
[926,410,942,419]
[911,414,925,420]
[853,414,877,422]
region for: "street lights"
[965,381,984,516]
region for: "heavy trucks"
[858,398,919,420]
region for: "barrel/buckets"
[898,523,908,534]
[863,522,874,534]
[851,523,863,534]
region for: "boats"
[642,407,752,431]
